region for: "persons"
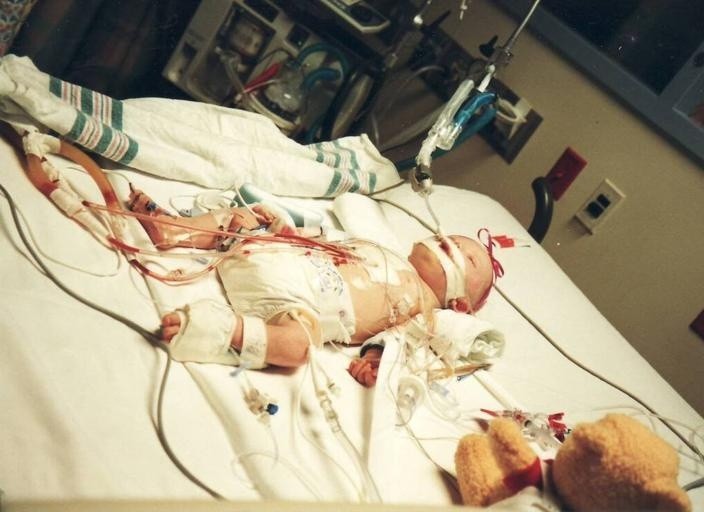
[129,189,495,386]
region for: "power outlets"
[406,25,544,166]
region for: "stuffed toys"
[457,411,690,511]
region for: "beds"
[0,54,703,512]
